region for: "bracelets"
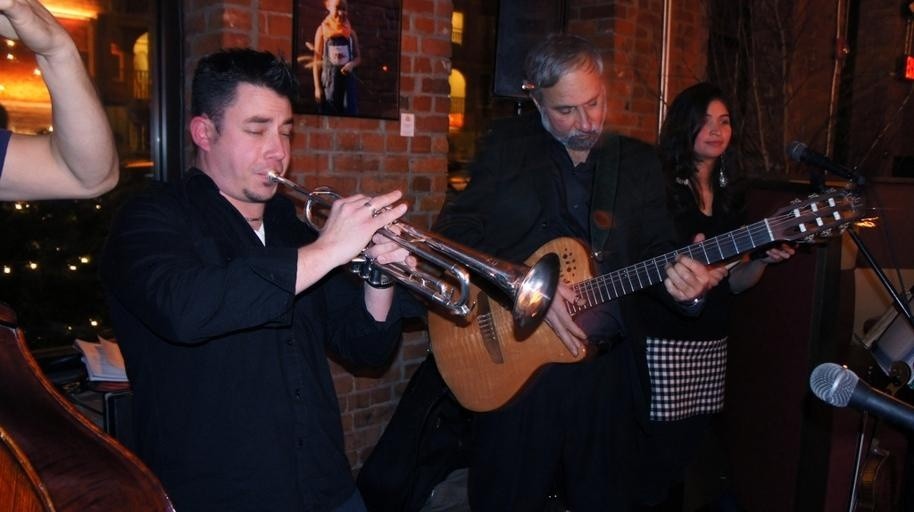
[364,278,394,289]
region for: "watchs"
[670,291,706,315]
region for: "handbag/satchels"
[352,348,476,512]
[640,326,725,426]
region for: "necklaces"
[241,215,263,222]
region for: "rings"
[679,284,690,292]
[572,294,582,308]
[363,201,377,218]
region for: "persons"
[311,0,363,117]
[645,81,800,511]
[0,1,122,203]
[96,45,417,510]
[428,29,733,510]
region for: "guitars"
[427,186,865,412]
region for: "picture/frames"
[291,0,403,121]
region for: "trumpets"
[266,168,558,327]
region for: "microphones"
[786,140,866,185]
[809,362,914,435]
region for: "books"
[69,332,131,387]
